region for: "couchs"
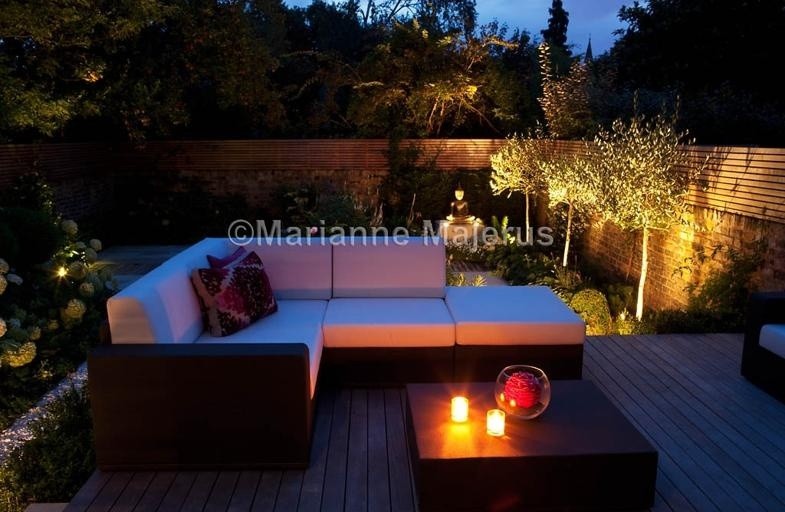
[89,286,586,472]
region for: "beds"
[406,381,658,511]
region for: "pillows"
[192,250,278,336]
[212,237,332,300]
[205,246,246,268]
[332,235,446,298]
[107,237,214,344]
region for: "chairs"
[741,291,785,405]
